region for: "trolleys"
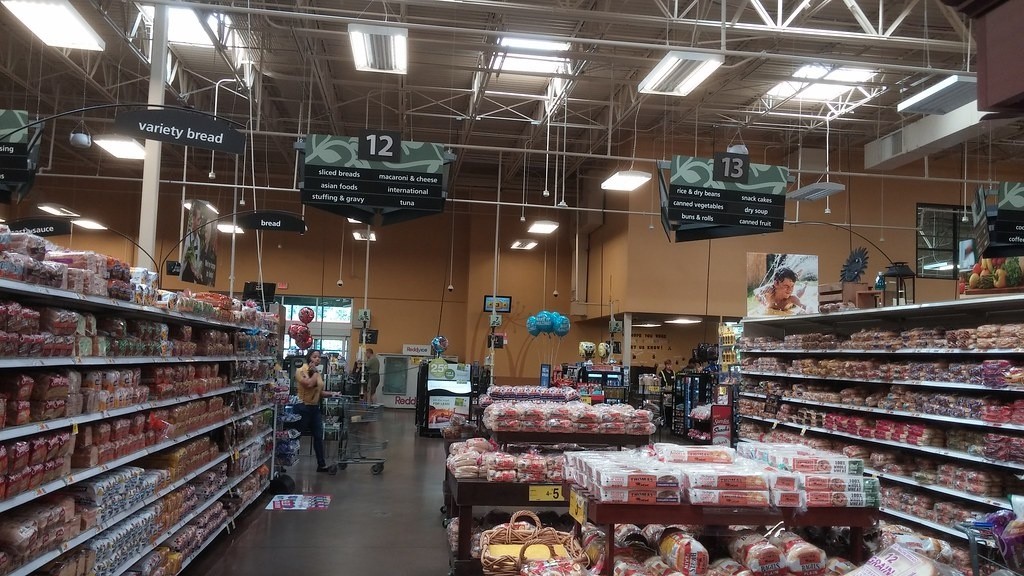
[953,520,1024,576]
[326,393,389,476]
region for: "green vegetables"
[979,256,1024,289]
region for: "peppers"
[990,269,1006,288]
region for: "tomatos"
[973,264,982,274]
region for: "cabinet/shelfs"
[817,282,868,308]
[0,278,288,576]
[630,386,665,443]
[738,293,1024,549]
[683,322,743,451]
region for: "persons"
[754,266,812,315]
[295,349,342,473]
[365,348,380,402]
[681,358,695,383]
[658,359,675,433]
[179,205,217,287]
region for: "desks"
[440,407,880,576]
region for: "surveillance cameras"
[553,291,559,297]
[337,280,343,287]
[447,285,454,292]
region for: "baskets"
[479,511,590,576]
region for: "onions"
[969,267,989,289]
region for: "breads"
[442,323,1024,576]
[0,224,279,576]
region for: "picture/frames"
[483,295,512,313]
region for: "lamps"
[638,0,725,97]
[785,166,846,201]
[348,0,409,74]
[0,0,105,52]
[883,262,916,307]
[509,238,538,250]
[184,184,245,233]
[526,209,559,233]
[898,0,978,116]
[37,158,109,230]
[352,229,376,241]
[91,134,145,160]
[601,102,652,191]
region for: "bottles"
[875,272,886,290]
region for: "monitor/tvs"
[484,295,512,314]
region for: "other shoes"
[317,466,335,475]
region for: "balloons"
[289,307,314,350]
[579,342,610,359]
[526,310,570,337]
